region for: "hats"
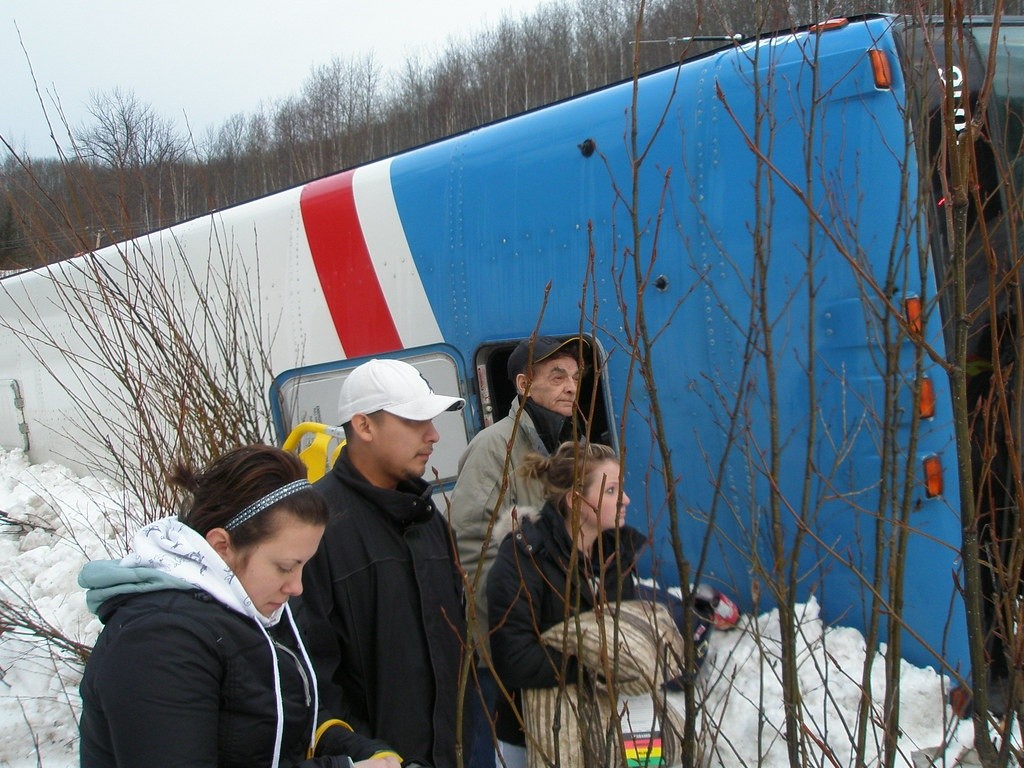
[338,359,466,427]
[508,336,590,380]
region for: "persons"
[78,444,421,768]
[300,358,481,768]
[444,335,648,768]
